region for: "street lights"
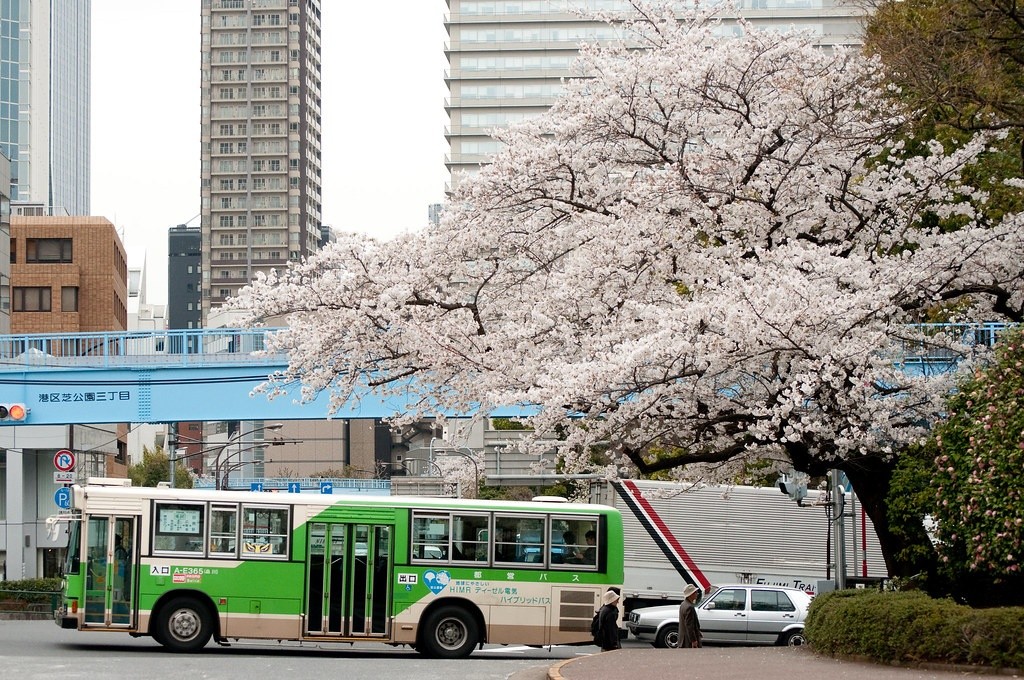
[214,422,285,530]
[435,449,478,499]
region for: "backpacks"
[590,611,599,638]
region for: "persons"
[495,539,501,560]
[593,590,622,652]
[115,533,127,563]
[677,584,704,649]
[562,530,596,565]
[441,535,462,560]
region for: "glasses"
[693,592,699,595]
[586,539,591,541]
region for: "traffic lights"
[0,403,31,422]
[776,479,809,502]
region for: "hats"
[115,534,122,540]
[683,584,700,599]
[603,590,620,605]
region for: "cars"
[626,584,815,649]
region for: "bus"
[45,478,627,659]
